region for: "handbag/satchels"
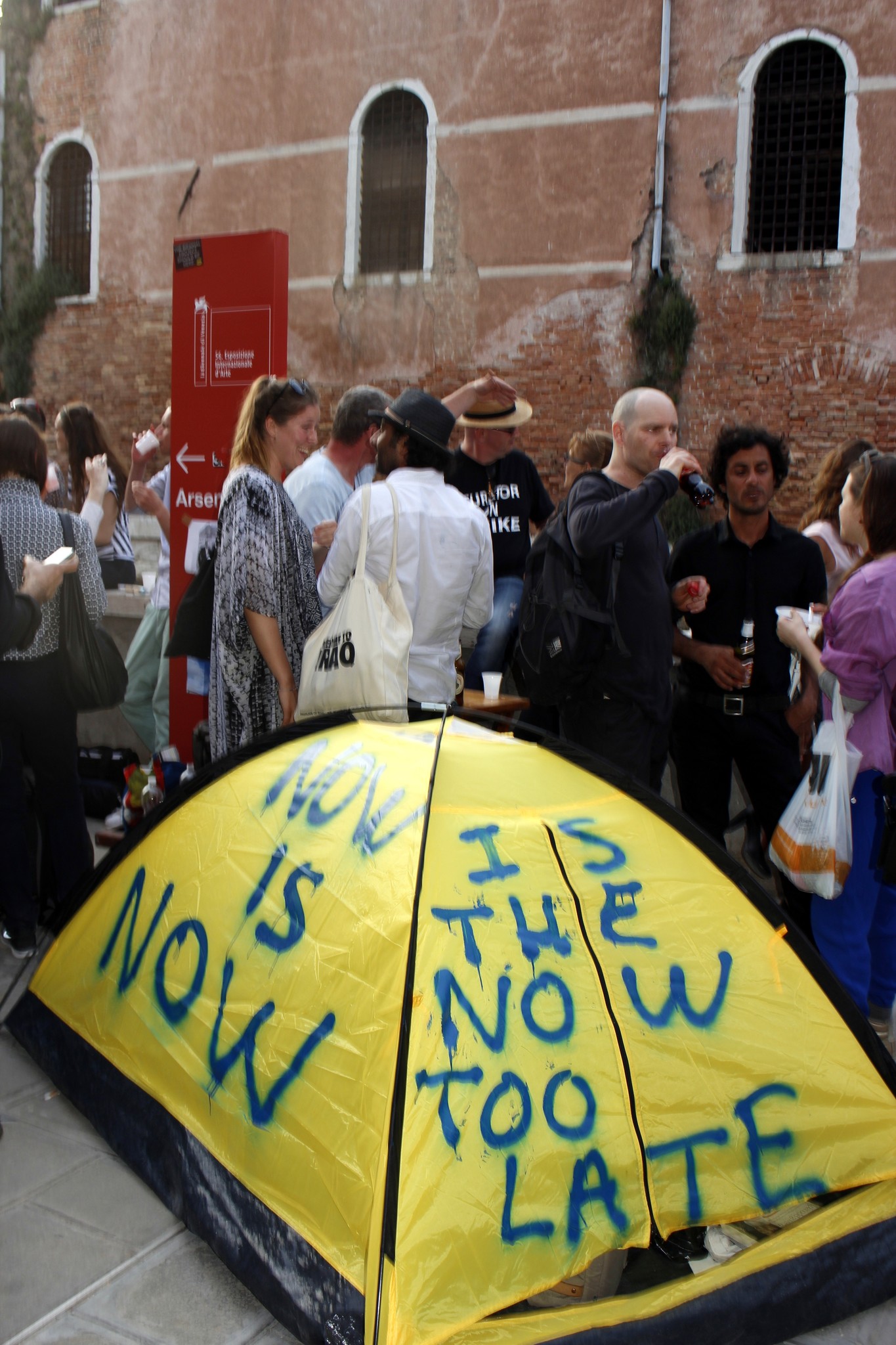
[163,546,215,660]
[768,681,863,900]
[871,773,896,888]
[294,571,414,724]
[56,507,129,708]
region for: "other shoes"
[104,807,130,830]
[866,1002,891,1041]
[1,925,38,960]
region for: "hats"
[456,396,533,428]
[369,387,456,457]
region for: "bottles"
[662,448,714,510]
[141,776,163,817]
[732,612,754,688]
[454,639,465,708]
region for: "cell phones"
[42,546,75,566]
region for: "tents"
[0,696,896,1345]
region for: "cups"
[482,671,502,700]
[135,429,160,455]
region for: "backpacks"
[511,469,620,712]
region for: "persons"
[520,384,710,809]
[284,383,399,585]
[317,387,495,712]
[786,441,880,755]
[0,537,80,664]
[414,375,557,691]
[4,397,68,511]
[666,425,829,883]
[775,448,895,1030]
[103,395,177,833]
[53,399,138,588]
[203,374,338,759]
[559,426,617,502]
[0,413,111,967]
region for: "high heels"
[725,805,771,880]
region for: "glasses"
[563,452,585,465]
[264,376,311,420]
[492,427,517,436]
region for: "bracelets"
[277,686,298,693]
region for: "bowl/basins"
[777,604,826,643]
